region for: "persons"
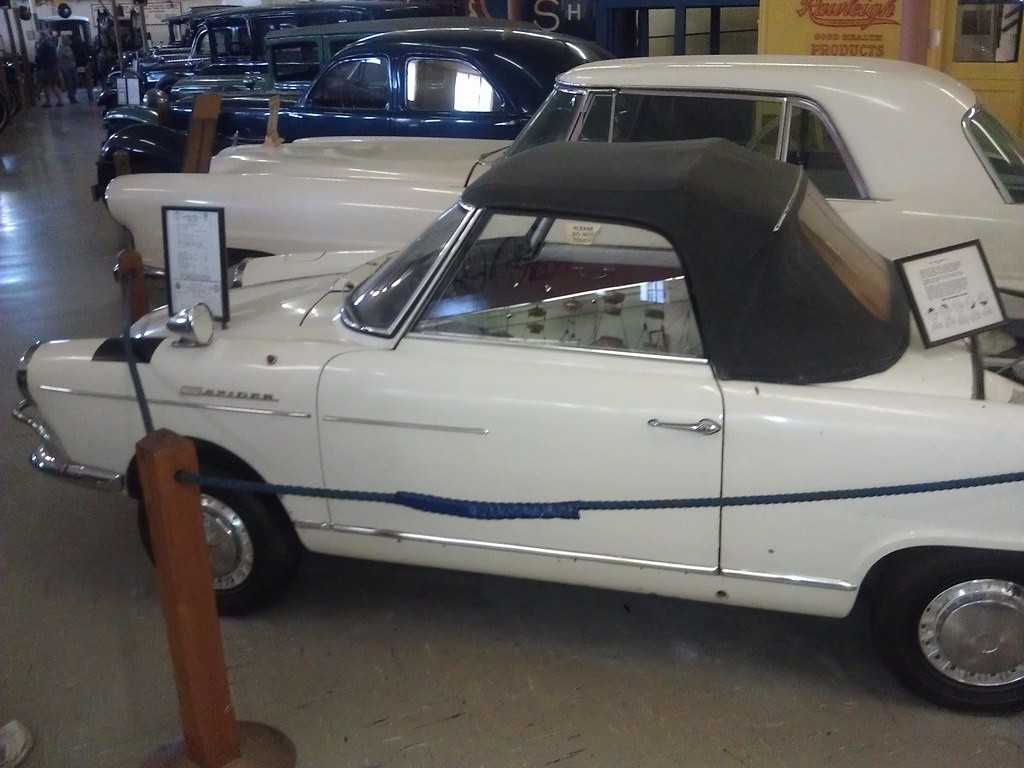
[56,35,80,103]
[35,28,66,106]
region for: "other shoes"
[70,99,78,103]
[88,94,93,100]
[55,102,62,106]
[42,103,51,107]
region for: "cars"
[9,134,1024,720]
[0,0,629,210]
[101,53,1024,294]
